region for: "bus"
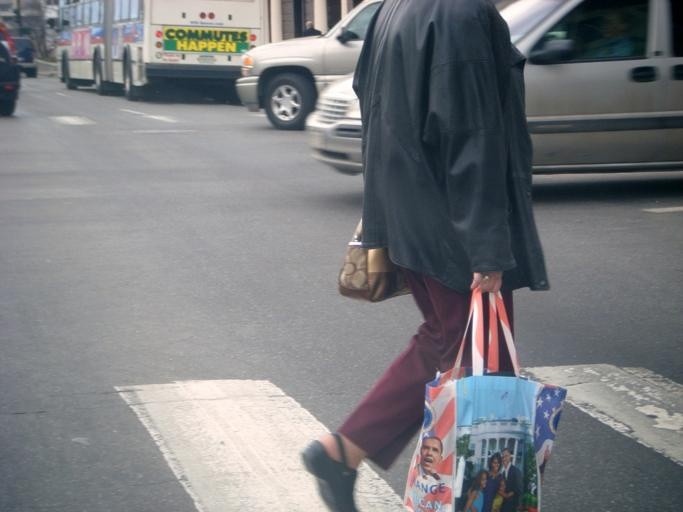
[44,1,268,101]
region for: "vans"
[301,0,683,179]
[0,19,38,116]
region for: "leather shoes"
[299,430,365,512]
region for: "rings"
[482,272,492,280]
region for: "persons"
[411,435,444,488]
[461,470,488,511]
[466,451,502,511]
[491,475,507,512]
[497,448,524,512]
[563,3,633,64]
[296,0,551,511]
[302,20,322,37]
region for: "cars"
[234,0,512,130]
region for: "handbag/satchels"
[402,279,571,512]
[334,210,415,304]
